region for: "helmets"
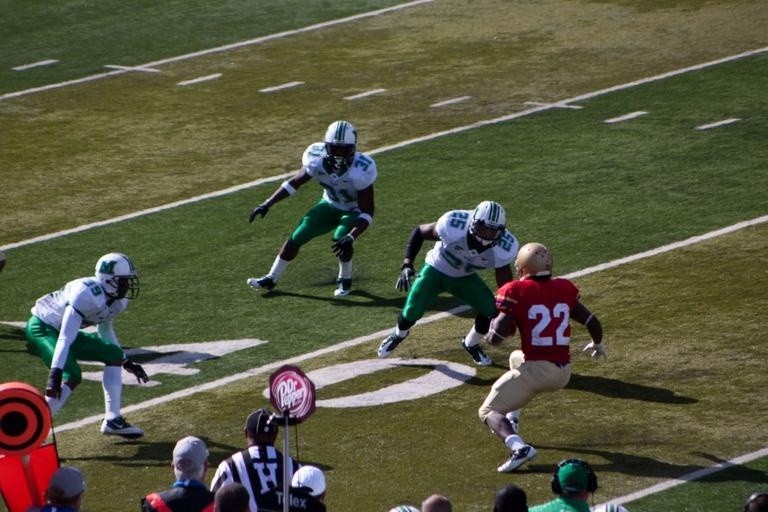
[322,121,357,170]
[469,201,506,247]
[514,243,553,280]
[95,253,140,299]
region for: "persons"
[24,465,86,512]
[25,251,151,438]
[213,481,251,512]
[491,484,529,512]
[244,119,379,298]
[139,435,215,512]
[528,458,600,512]
[212,407,303,511]
[422,493,453,512]
[377,200,520,366]
[478,242,604,473]
[261,465,329,512]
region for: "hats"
[292,465,326,496]
[50,467,85,496]
[557,458,591,493]
[172,436,210,472]
[246,408,278,434]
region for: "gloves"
[249,204,269,223]
[122,358,149,383]
[583,341,607,363]
[395,263,420,292]
[331,235,353,259]
[45,368,64,400]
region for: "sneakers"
[490,418,519,435]
[497,445,537,473]
[247,276,277,292]
[462,336,492,366]
[378,331,409,358]
[100,417,143,439]
[334,278,352,297]
